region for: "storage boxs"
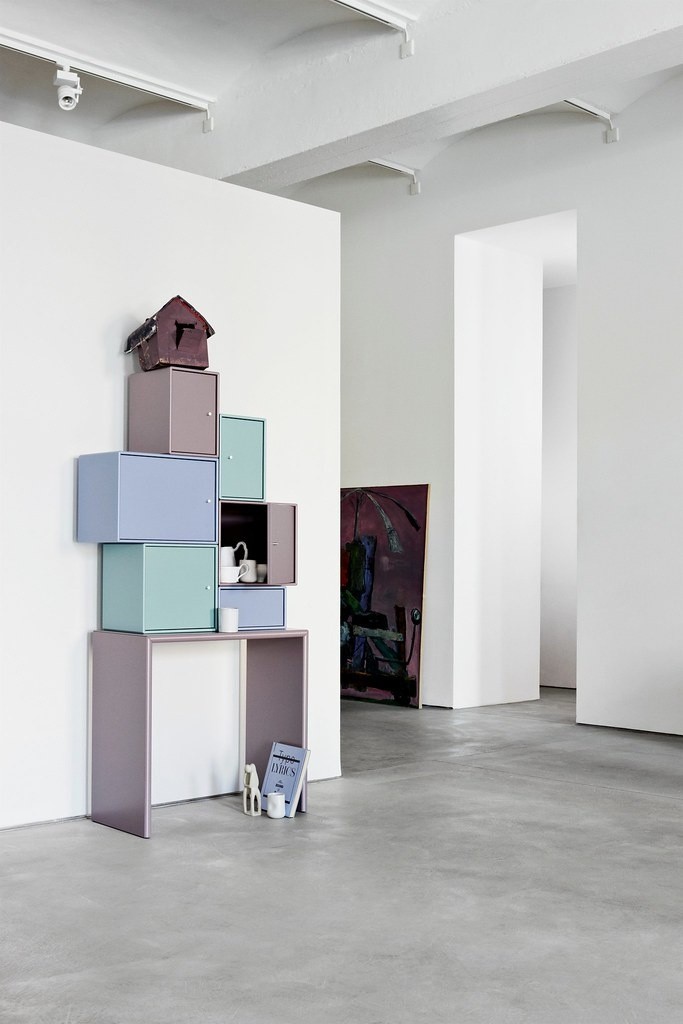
[216,414,267,501]
[128,368,220,456]
[76,452,218,544]
[102,543,217,635]
[218,586,287,631]
[220,501,296,586]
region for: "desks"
[90,630,310,838]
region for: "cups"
[268,793,286,818]
[240,560,256,582]
[220,542,248,568]
[218,607,239,632]
[219,564,249,584]
[256,564,267,582]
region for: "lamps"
[53,64,83,109]
